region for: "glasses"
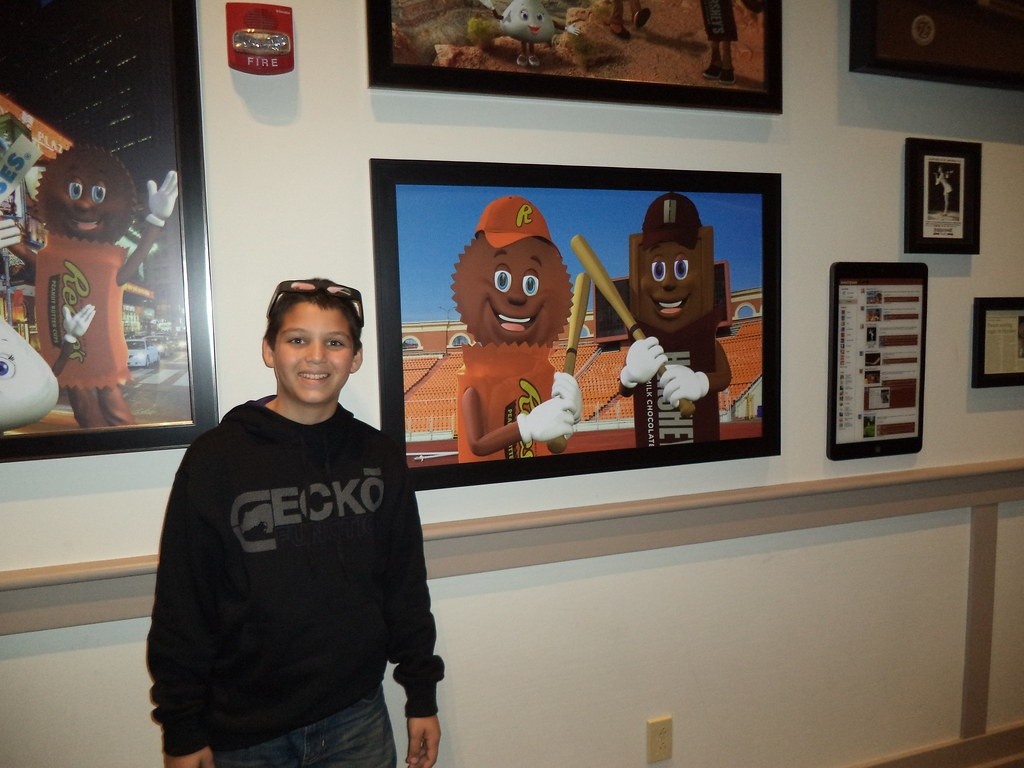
[267,280,364,327]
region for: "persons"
[934,165,953,216]
[145,279,448,768]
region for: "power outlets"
[647,714,674,765]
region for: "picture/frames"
[368,152,786,486]
[363,0,786,120]
[824,261,934,465]
[970,296,1024,390]
[843,0,1024,97]
[0,0,223,462]
[904,135,986,256]
